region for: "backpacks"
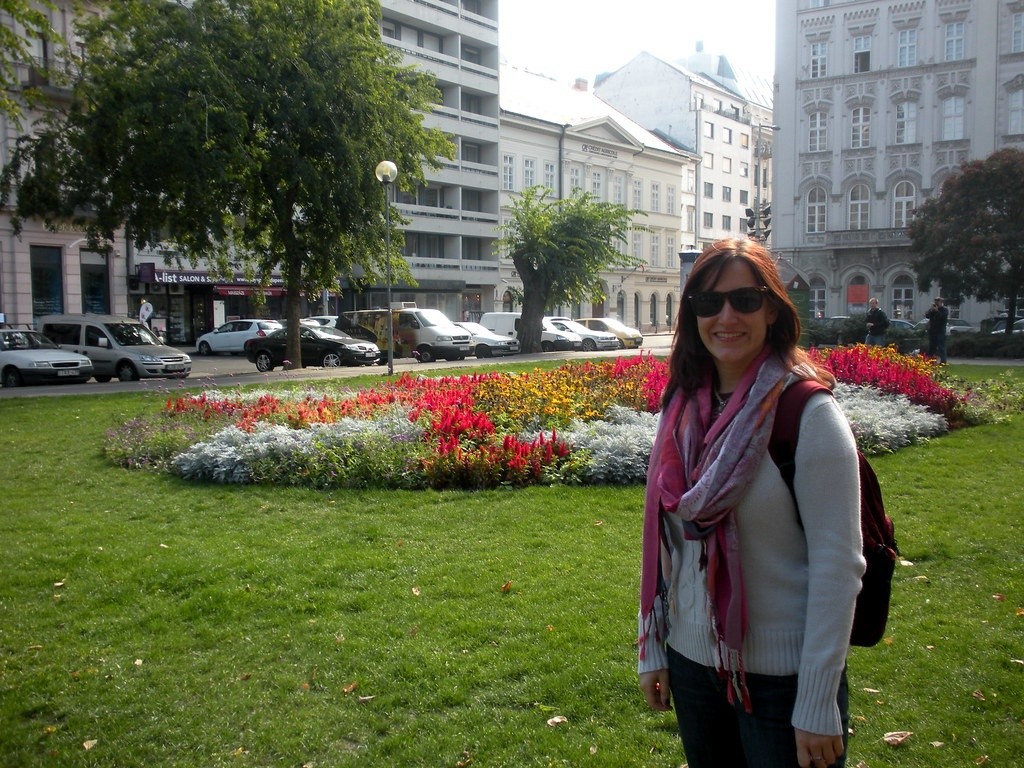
[768,377,899,647]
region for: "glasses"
[688,286,774,318]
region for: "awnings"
[217,285,288,296]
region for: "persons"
[865,298,890,346]
[639,237,867,768]
[926,297,949,365]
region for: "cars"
[0,329,94,388]
[891,319,915,328]
[196,319,283,356]
[551,321,620,352]
[810,317,851,347]
[913,319,974,334]
[308,315,339,327]
[278,318,319,326]
[991,319,1024,334]
[452,321,521,360]
[244,326,380,373]
[575,318,643,350]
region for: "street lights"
[376,160,398,374]
[745,123,782,242]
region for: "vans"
[479,312,582,353]
[36,313,192,382]
[334,302,474,363]
